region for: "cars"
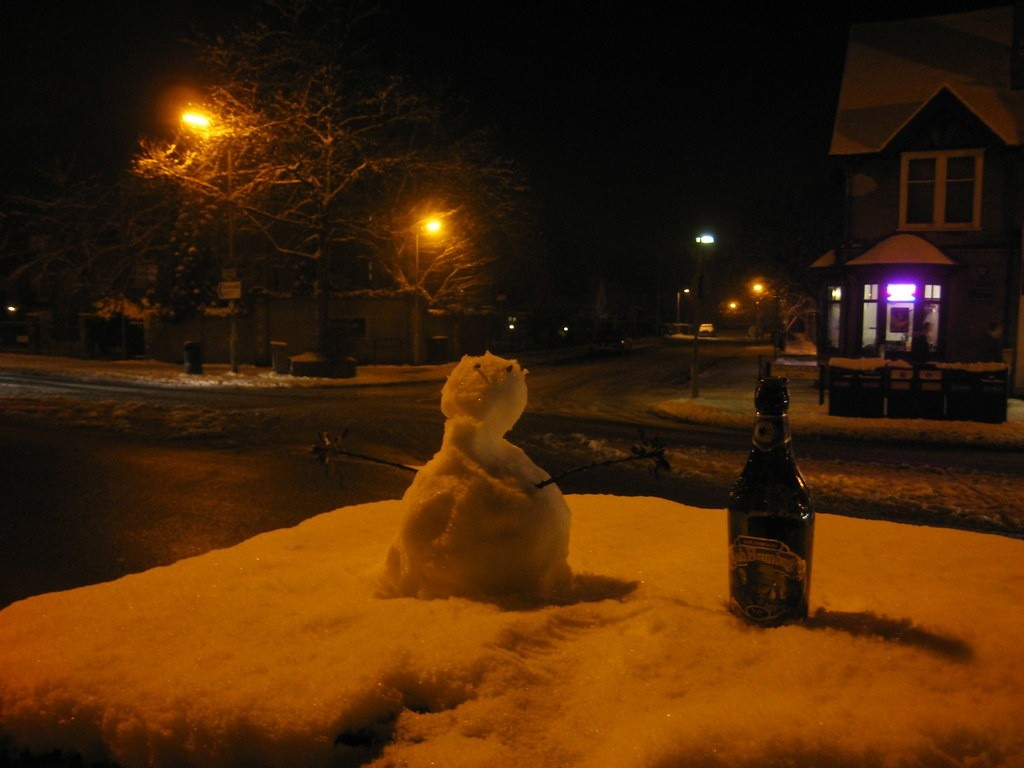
[699,323,716,338]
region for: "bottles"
[724,376,815,627]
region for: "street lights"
[691,231,716,383]
[412,212,447,365]
[177,106,242,374]
[751,282,764,346]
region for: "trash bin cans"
[184,340,204,374]
[270,342,289,374]
